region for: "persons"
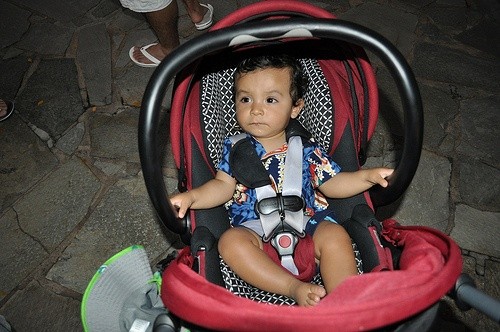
[120,0,215,67]
[170,52,394,307]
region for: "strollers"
[129,0,500,332]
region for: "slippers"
[195,3,214,30]
[0,98,14,123]
[129,43,161,67]
